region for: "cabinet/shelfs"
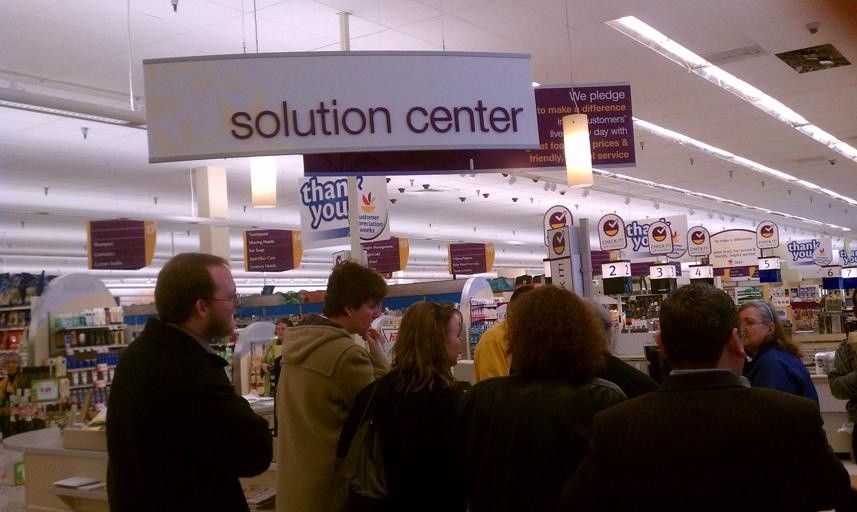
[722,280,857,457]
[370,278,670,377]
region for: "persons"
[735,298,822,416]
[332,300,470,511]
[445,285,630,511]
[104,252,275,512]
[554,281,853,509]
[826,285,857,467]
[0,350,32,407]
[270,259,395,512]
[580,297,657,398]
[472,285,533,384]
[260,317,295,397]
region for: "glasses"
[209,294,241,308]
[737,330,745,340]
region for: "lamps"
[250,3,277,208]
[561,1,594,192]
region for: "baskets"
[799,348,838,366]
